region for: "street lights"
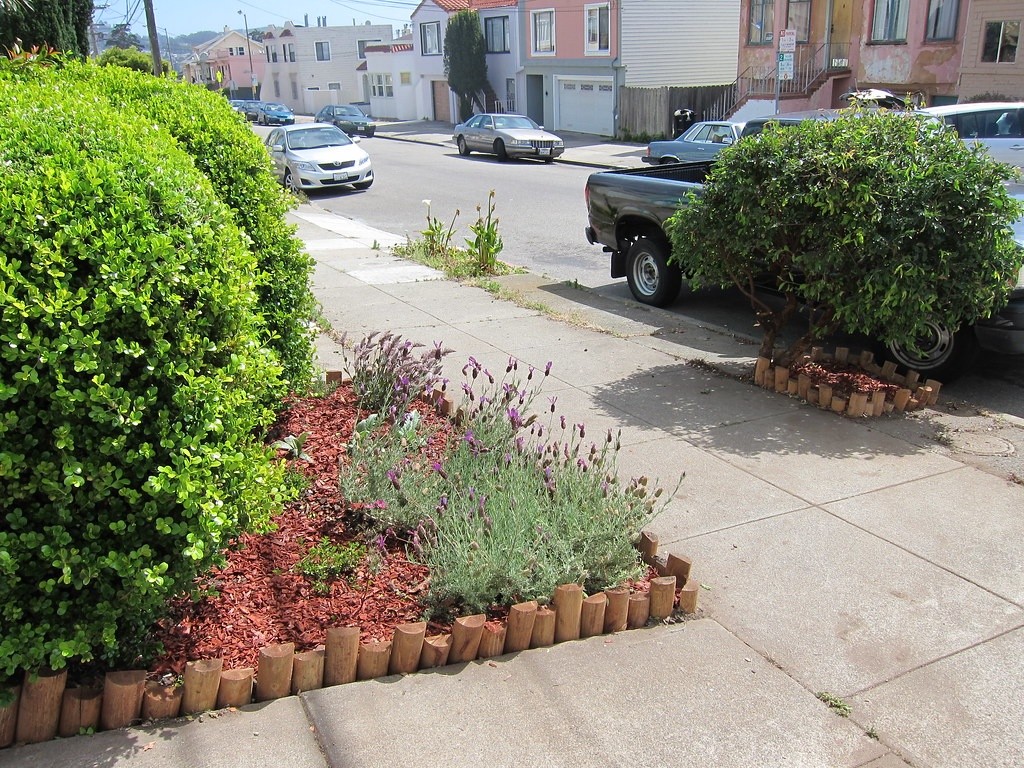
[238,10,255,100]
[142,24,174,70]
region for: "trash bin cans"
[673,108,695,139]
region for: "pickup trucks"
[584,110,1024,382]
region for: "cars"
[640,121,746,165]
[839,88,1024,183]
[314,105,376,138]
[452,114,564,163]
[230,100,243,111]
[262,123,374,195]
[238,100,266,122]
[258,102,296,126]
